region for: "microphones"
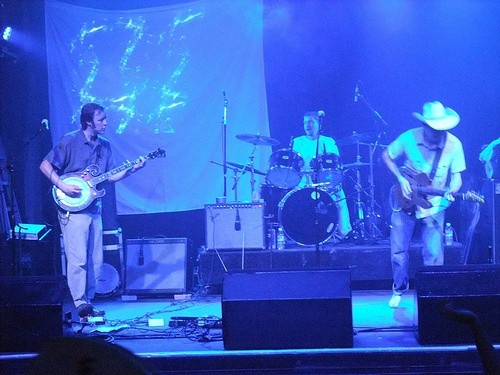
[317,110,326,116]
[139,246,145,265]
[355,82,360,102]
[235,210,241,230]
[40,119,49,130]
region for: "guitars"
[393,165,487,211]
[52,147,167,213]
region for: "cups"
[252,191,260,202]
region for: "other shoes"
[77,303,94,318]
[389,294,401,308]
[89,306,106,317]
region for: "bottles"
[445,223,453,245]
[277,227,285,250]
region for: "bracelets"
[130,168,134,173]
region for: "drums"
[278,186,340,248]
[267,148,305,190]
[309,152,344,188]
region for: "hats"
[413,100,462,131]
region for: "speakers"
[205,202,266,251]
[221,266,354,349]
[415,178,500,346]
[126,237,194,297]
[0,303,63,353]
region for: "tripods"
[331,94,390,246]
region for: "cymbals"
[336,132,373,147]
[226,161,266,177]
[235,134,282,147]
[345,161,376,169]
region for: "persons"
[381,100,466,308]
[40,103,147,317]
[292,112,352,238]
